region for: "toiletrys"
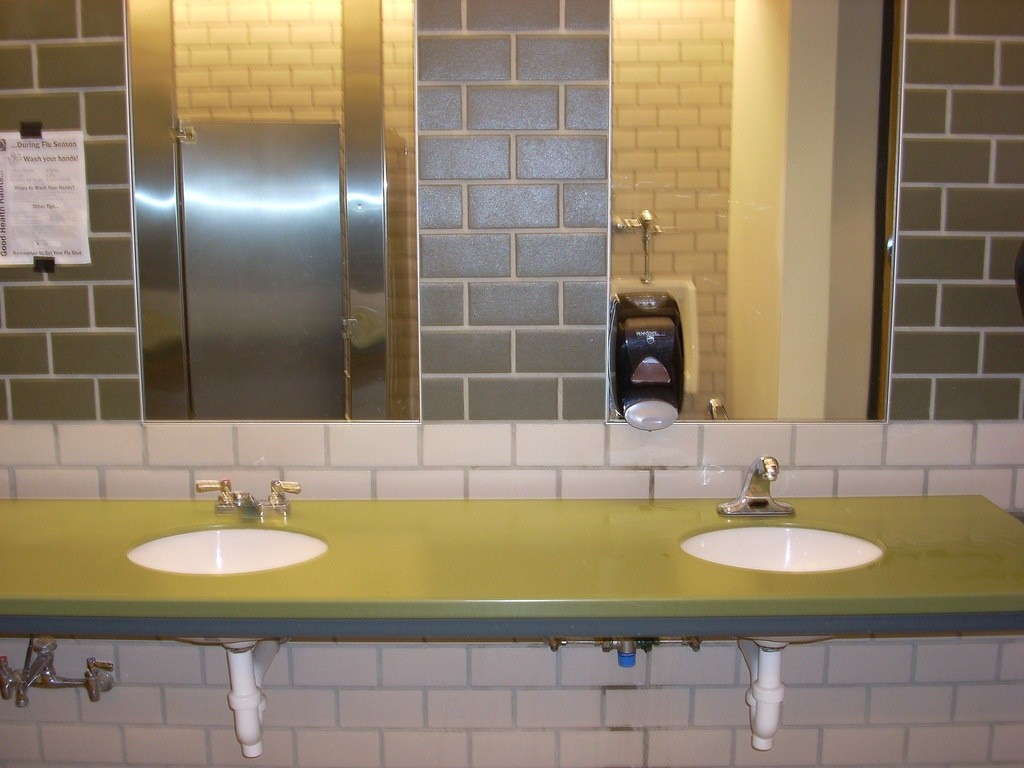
[606,293,684,430]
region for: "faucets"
[232,491,263,522]
[707,397,730,422]
[740,453,779,516]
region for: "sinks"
[124,527,331,578]
[678,524,886,574]
